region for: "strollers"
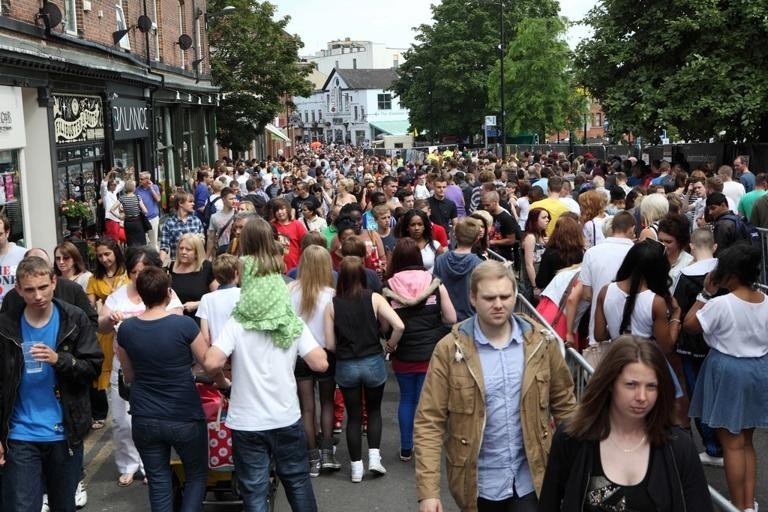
[169,375,282,512]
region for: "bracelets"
[695,287,713,303]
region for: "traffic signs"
[487,129,503,137]
[485,116,496,126]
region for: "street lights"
[415,65,435,146]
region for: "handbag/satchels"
[207,420,235,473]
[535,294,569,343]
[140,212,151,233]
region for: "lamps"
[168,89,217,106]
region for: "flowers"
[61,196,94,216]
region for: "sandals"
[92,418,105,430]
[117,474,135,485]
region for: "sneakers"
[699,451,724,467]
[310,448,386,482]
[75,478,86,508]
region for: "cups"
[20,340,44,374]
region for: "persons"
[0,257,106,512]
[115,265,232,512]
[538,330,715,511]
[409,258,579,512]
[681,240,767,512]
[1,138,767,488]
[203,314,331,511]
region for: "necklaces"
[608,435,645,454]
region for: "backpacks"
[201,195,223,231]
[716,211,752,242]
